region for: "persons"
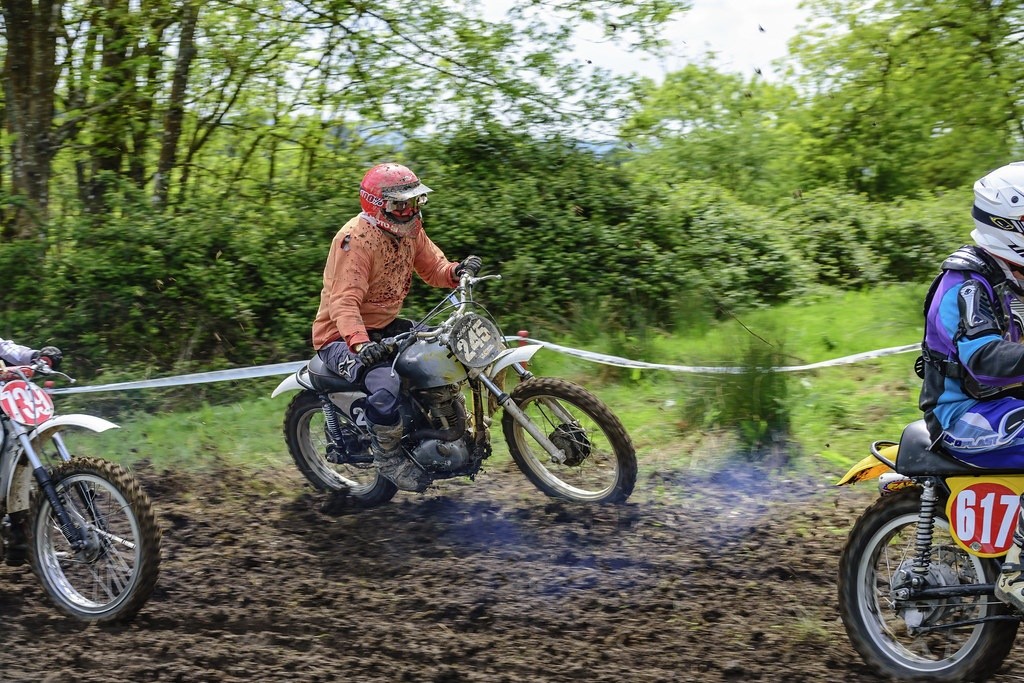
[311,162,492,491]
[0,336,63,566]
[919,160,1024,611]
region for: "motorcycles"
[838,418,1024,683]
[272,260,639,506]
[0,354,163,624]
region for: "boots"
[363,409,422,491]
[993,493,1023,613]
[483,415,493,429]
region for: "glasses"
[396,193,429,211]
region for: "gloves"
[357,341,385,368]
[39,346,63,369]
[455,255,482,278]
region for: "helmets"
[967,157,1022,269]
[358,161,423,239]
